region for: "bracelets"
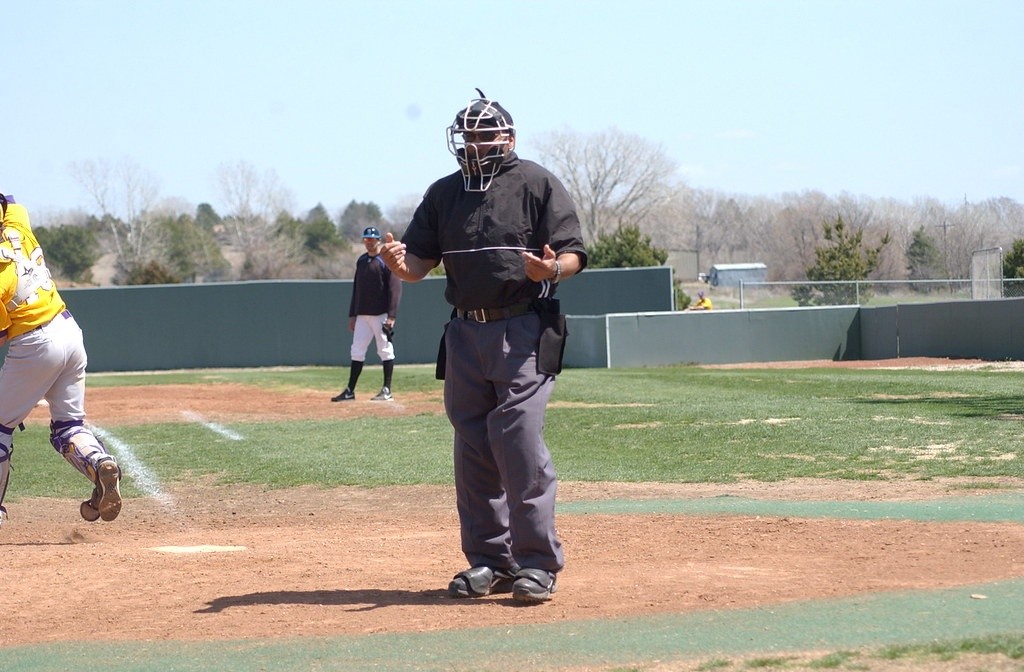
[548,261,562,285]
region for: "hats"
[451,102,514,133]
[362,226,380,240]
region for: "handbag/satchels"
[537,307,568,374]
[435,321,451,380]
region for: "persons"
[0,191,122,522]
[378,87,589,604]
[688,291,713,310]
[330,227,402,402]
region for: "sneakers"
[371,392,393,401]
[331,389,355,402]
[98,461,122,521]
[513,567,558,601]
[448,566,515,597]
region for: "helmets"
[445,99,516,191]
